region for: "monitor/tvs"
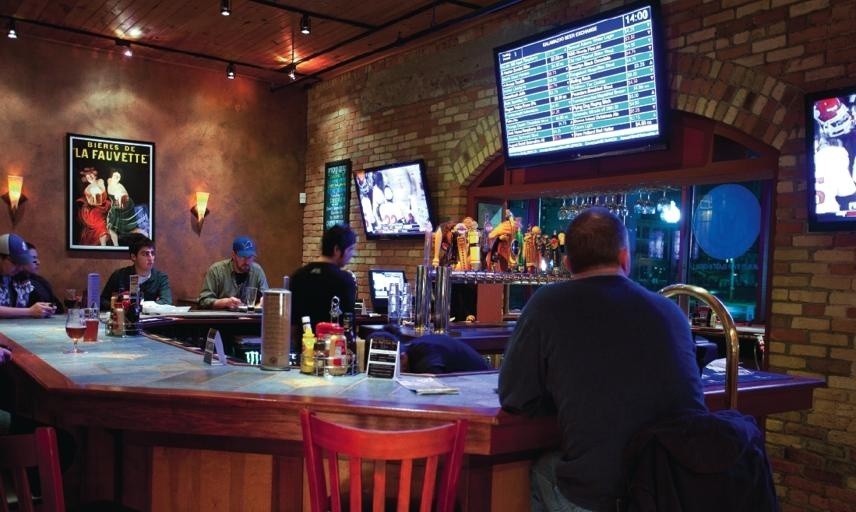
[353,158,436,239]
[368,270,407,314]
[805,86,856,231]
[493,0,668,171]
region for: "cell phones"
[49,302,57,306]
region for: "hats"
[233,237,257,258]
[0,233,32,265]
[366,331,408,353]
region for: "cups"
[387,281,414,326]
[245,286,258,314]
[82,308,99,341]
[63,289,76,310]
[434,265,451,335]
[413,265,432,332]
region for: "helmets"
[355,175,371,194]
[372,171,383,181]
[813,97,853,138]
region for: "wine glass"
[65,310,88,356]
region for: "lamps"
[6,14,237,80]
[1,175,29,221]
[219,0,312,37]
[190,190,209,229]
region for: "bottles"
[300,311,365,375]
[260,288,291,371]
[282,276,289,288]
[752,336,766,369]
[104,287,143,337]
[692,301,716,328]
[86,273,99,317]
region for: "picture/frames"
[64,132,156,261]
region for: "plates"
[159,304,192,315]
[239,305,262,312]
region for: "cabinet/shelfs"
[176,297,199,310]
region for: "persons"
[400,333,490,373]
[362,184,431,233]
[75,166,112,245]
[497,205,712,512]
[197,235,270,311]
[100,238,172,311]
[104,168,149,247]
[813,95,856,223]
[0,232,58,319]
[287,223,359,366]
[0,347,13,434]
[8,241,64,315]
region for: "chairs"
[0,421,65,511]
[297,404,470,511]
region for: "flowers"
[442,216,479,261]
[531,226,566,256]
